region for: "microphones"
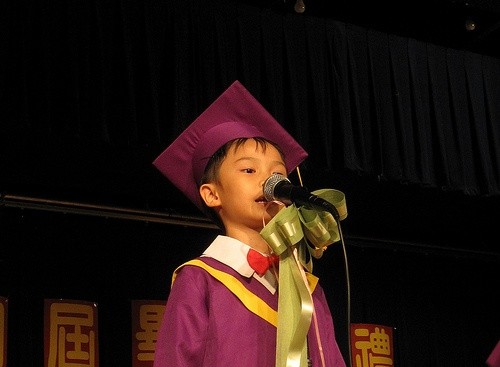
[263,175,339,220]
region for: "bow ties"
[247,248,279,278]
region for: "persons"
[152,81,347,367]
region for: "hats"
[152,79,309,230]
